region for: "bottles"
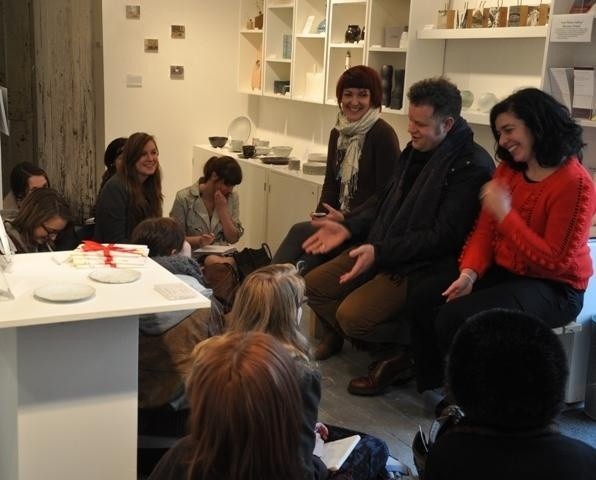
[398,25,408,50]
[344,51,351,70]
[246,10,262,31]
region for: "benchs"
[307,305,582,339]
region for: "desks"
[0,250,211,480]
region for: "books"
[72,242,149,269]
[312,433,361,471]
[194,245,237,256]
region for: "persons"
[75,138,127,238]
[412,89,595,416]
[225,264,389,477]
[270,66,401,359]
[1,162,30,210]
[416,308,595,478]
[132,217,226,436]
[1,187,72,255]
[170,155,243,267]
[96,134,192,258]
[13,167,79,251]
[149,332,325,479]
[301,77,496,396]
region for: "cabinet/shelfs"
[193,142,323,260]
[236,1,595,129]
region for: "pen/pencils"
[194,228,203,234]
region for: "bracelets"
[460,272,475,284]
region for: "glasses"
[40,223,62,235]
[297,296,310,309]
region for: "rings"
[321,245,326,249]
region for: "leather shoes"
[312,333,344,360]
[348,349,415,396]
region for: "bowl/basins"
[207,137,227,148]
[253,146,270,155]
[254,141,268,146]
[270,145,292,158]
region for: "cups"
[230,140,243,152]
[252,138,259,145]
[287,159,300,171]
[241,145,256,158]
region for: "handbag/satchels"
[233,243,272,281]
[437,0,550,29]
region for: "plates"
[32,283,94,303]
[226,115,251,146]
[260,157,288,165]
[86,268,141,284]
